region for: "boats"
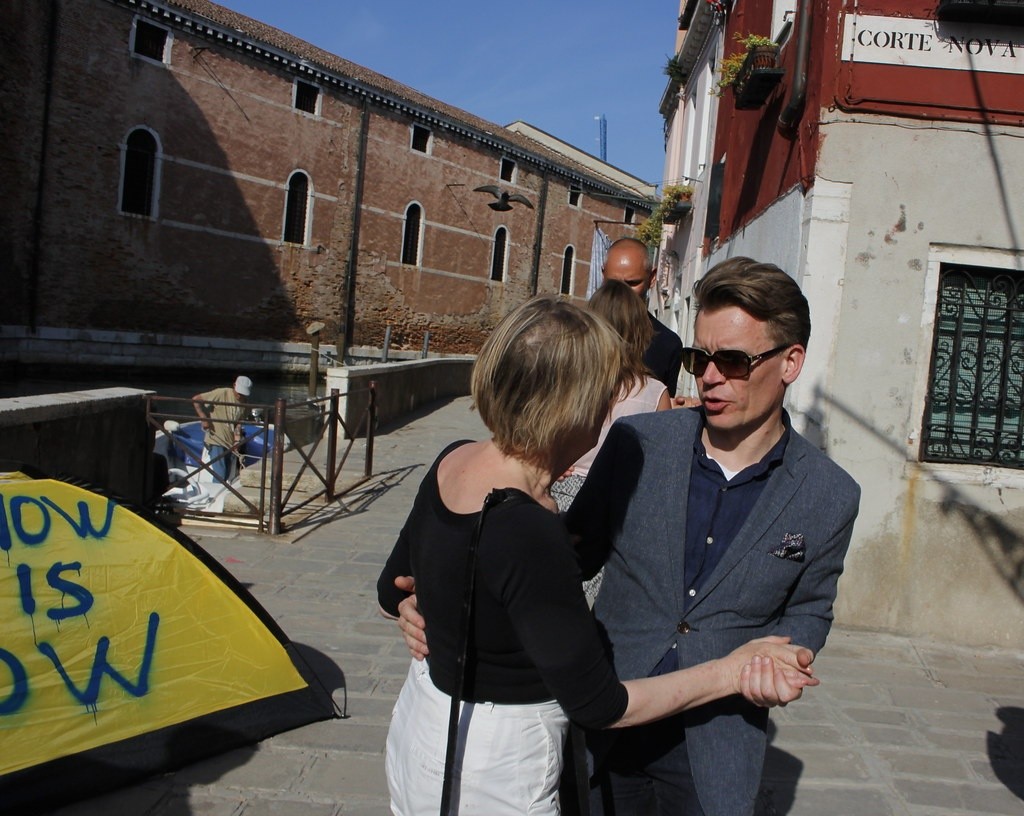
[154,419,291,509]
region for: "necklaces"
[521,461,548,494]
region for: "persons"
[558,238,703,481]
[375,295,819,816]
[550,280,700,610]
[394,256,860,816]
[192,376,252,483]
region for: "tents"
[0,469,344,816]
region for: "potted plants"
[706,26,780,99]
[635,183,693,248]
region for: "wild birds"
[472,185,534,211]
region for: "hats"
[234,375,253,397]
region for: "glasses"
[679,342,790,381]
